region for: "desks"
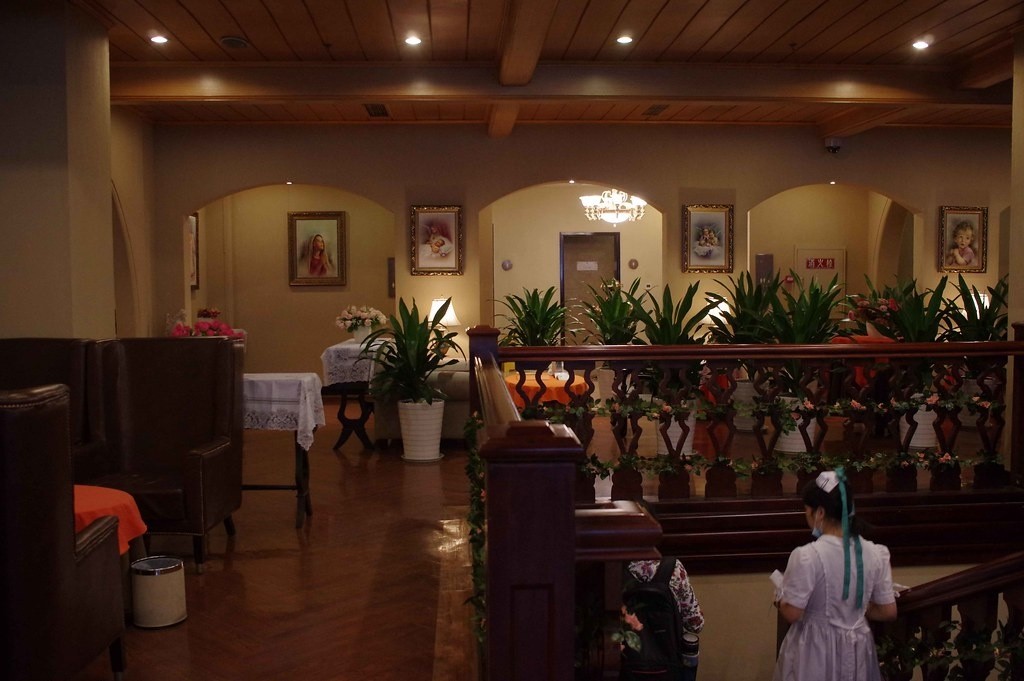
[240,373,326,529]
[831,333,896,385]
[504,370,587,410]
[320,339,391,455]
[74,483,148,613]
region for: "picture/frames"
[286,210,348,287]
[188,212,200,291]
[939,204,988,274]
[409,203,464,276]
[679,203,734,275]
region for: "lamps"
[427,298,463,357]
[578,188,647,227]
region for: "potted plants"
[590,274,1009,450]
[355,296,466,464]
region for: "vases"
[354,321,374,345]
[866,321,883,338]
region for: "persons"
[773,465,898,681]
[625,558,705,681]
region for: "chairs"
[0,384,147,681]
[69,338,247,576]
[0,339,87,446]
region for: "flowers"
[530,392,994,476]
[611,603,646,654]
[166,317,247,340]
[336,304,391,331]
[844,290,902,328]
[461,411,489,643]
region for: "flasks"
[682,633,699,681]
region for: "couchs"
[374,339,468,443]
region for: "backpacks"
[616,555,683,679]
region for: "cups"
[551,361,564,376]
